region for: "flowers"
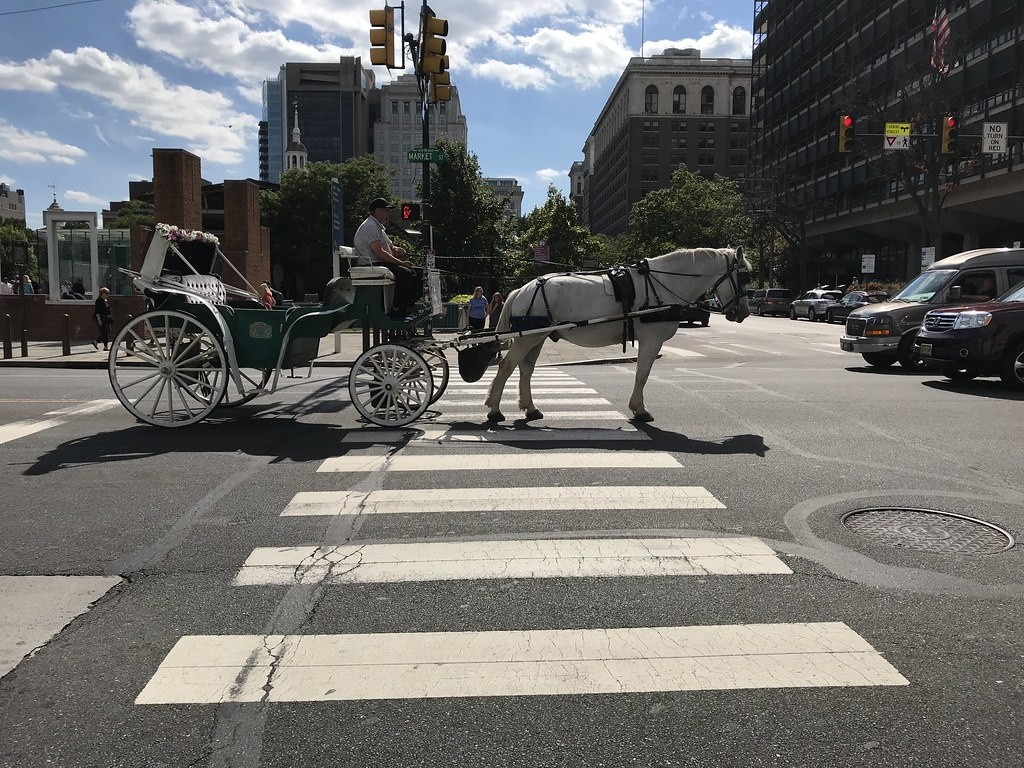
[154,222,220,256]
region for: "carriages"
[105,196,754,425]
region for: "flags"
[931,0,951,75]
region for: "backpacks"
[271,289,283,306]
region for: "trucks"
[839,247,1024,366]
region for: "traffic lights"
[839,116,853,152]
[941,116,957,153]
[430,55,451,101]
[368,9,394,65]
[420,13,448,74]
[401,204,420,221]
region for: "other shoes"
[92,341,99,350]
[103,348,110,351]
[389,308,413,323]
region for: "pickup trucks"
[910,280,1024,393]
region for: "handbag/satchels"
[105,315,115,324]
[463,325,471,331]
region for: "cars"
[826,291,888,325]
[704,293,722,311]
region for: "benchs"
[339,245,396,286]
[285,276,357,326]
[178,271,229,306]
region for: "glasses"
[104,292,111,296]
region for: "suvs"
[789,290,842,322]
[748,289,794,316]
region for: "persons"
[259,280,276,309]
[0,274,85,300]
[962,278,994,301]
[467,287,489,347]
[353,199,418,321]
[489,292,505,328]
[92,287,114,351]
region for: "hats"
[369,198,397,213]
[23,275,32,283]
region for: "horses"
[483,244,753,424]
